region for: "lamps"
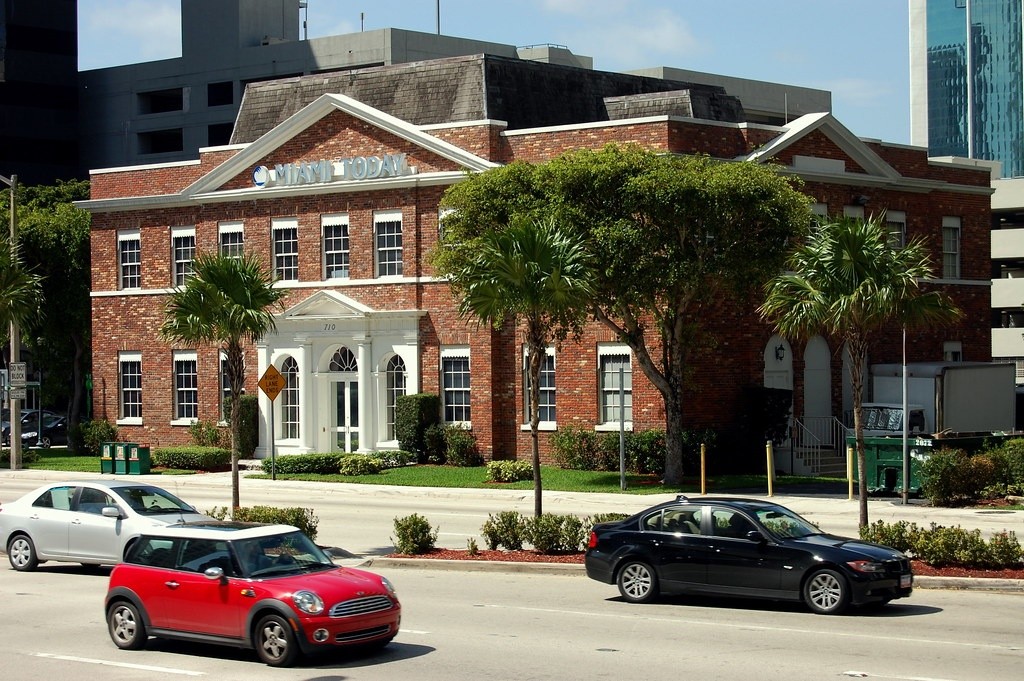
[775,344,785,361]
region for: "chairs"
[184,540,232,573]
[146,548,172,567]
[714,512,756,536]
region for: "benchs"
[648,513,700,534]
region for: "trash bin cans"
[99,441,151,475]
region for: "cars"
[0,478,222,574]
[1,409,52,444]
[102,518,401,665]
[585,494,915,615]
[8,413,95,449]
